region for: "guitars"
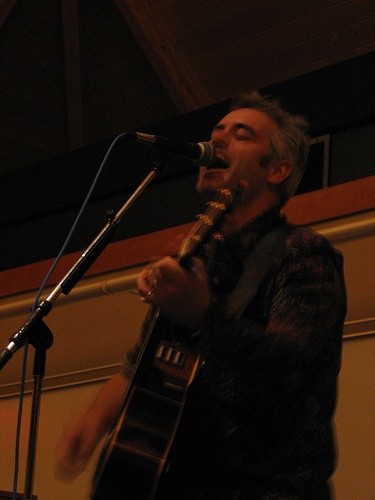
[89,189,229,500]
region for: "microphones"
[127,131,216,168]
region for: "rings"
[146,288,153,298]
[55,91,349,500]
[149,279,159,289]
